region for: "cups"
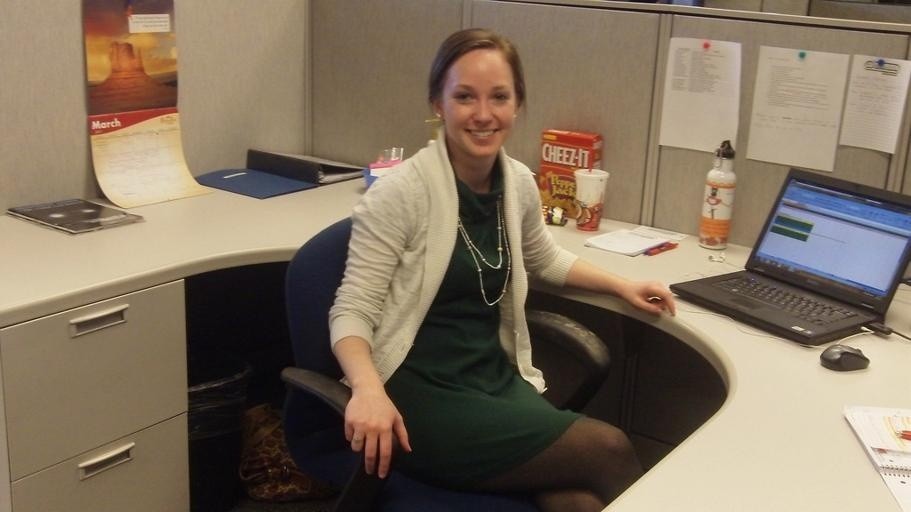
[382,146,403,161]
[573,167,610,233]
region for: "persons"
[327,28,677,512]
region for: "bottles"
[698,138,738,249]
[540,204,568,227]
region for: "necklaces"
[457,195,510,306]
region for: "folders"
[247,148,370,186]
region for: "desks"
[0,155,911,511]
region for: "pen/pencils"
[648,242,679,256]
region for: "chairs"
[276,210,616,511]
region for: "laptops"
[669,167,911,345]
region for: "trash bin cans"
[187,355,252,512]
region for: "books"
[6,199,144,234]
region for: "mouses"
[820,345,869,371]
